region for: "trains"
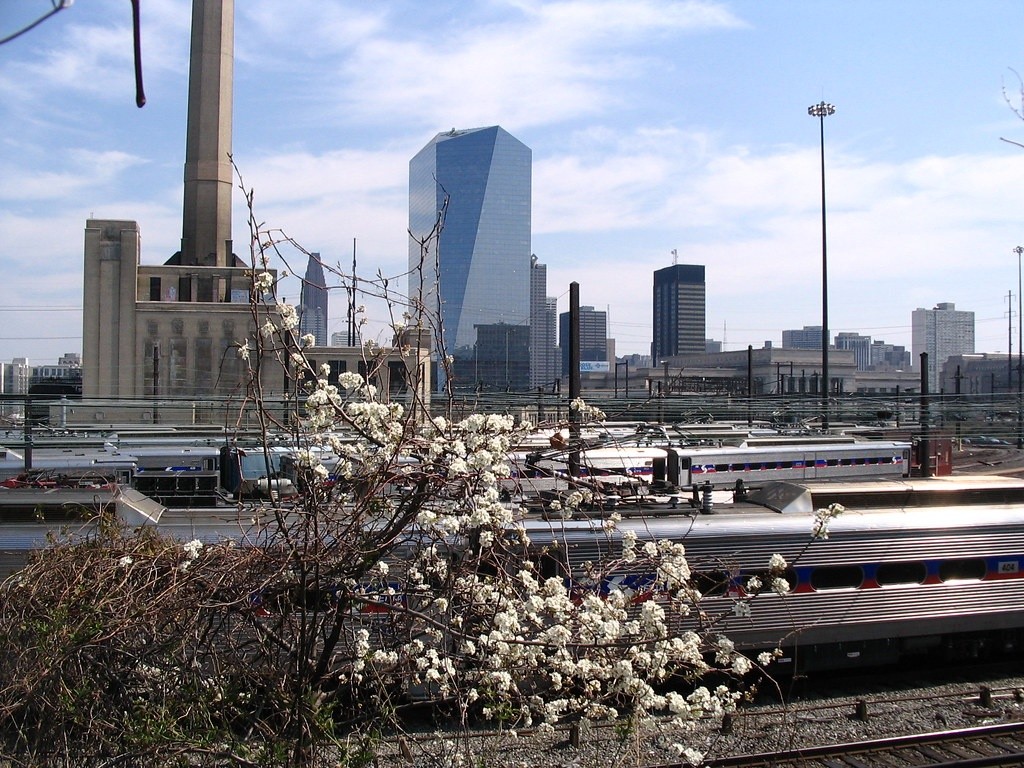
[0,439,1023,768]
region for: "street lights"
[808,99,838,432]
[1013,245,1024,394]
